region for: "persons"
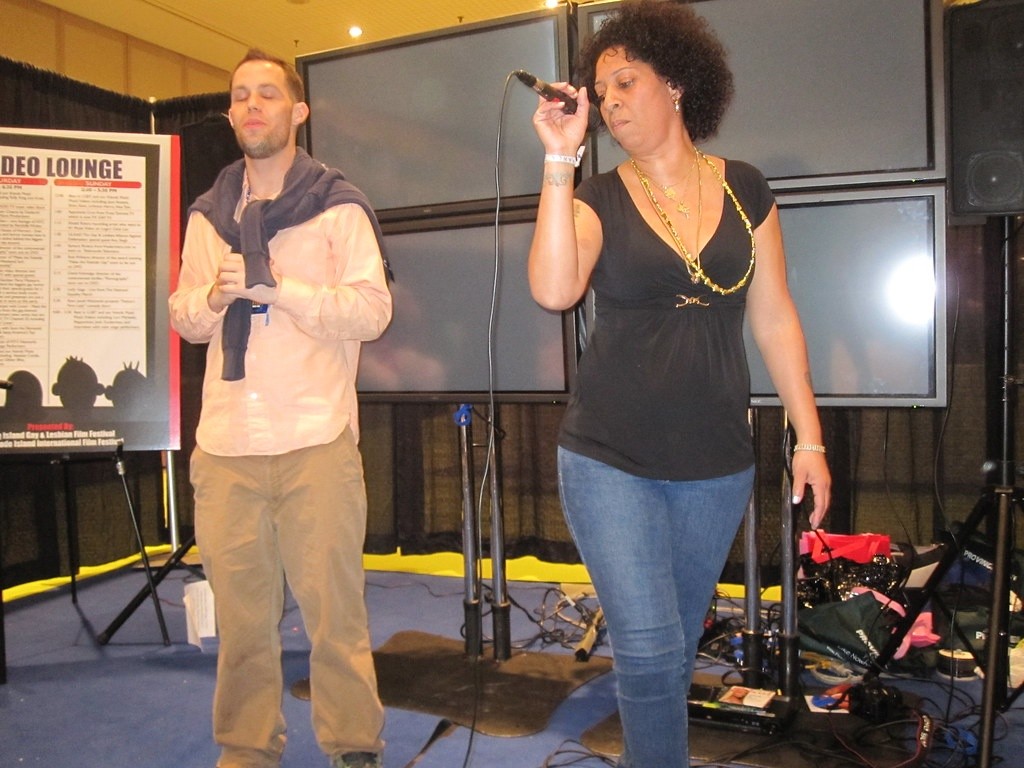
[170,47,396,768]
[528,0,832,768]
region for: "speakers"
[180,113,244,214]
[943,0,1024,218]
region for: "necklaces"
[630,146,755,294]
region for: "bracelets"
[794,443,825,453]
[544,146,585,166]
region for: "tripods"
[861,213,1024,712]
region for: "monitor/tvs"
[295,0,947,404]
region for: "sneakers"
[329,750,385,768]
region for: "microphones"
[516,70,602,132]
